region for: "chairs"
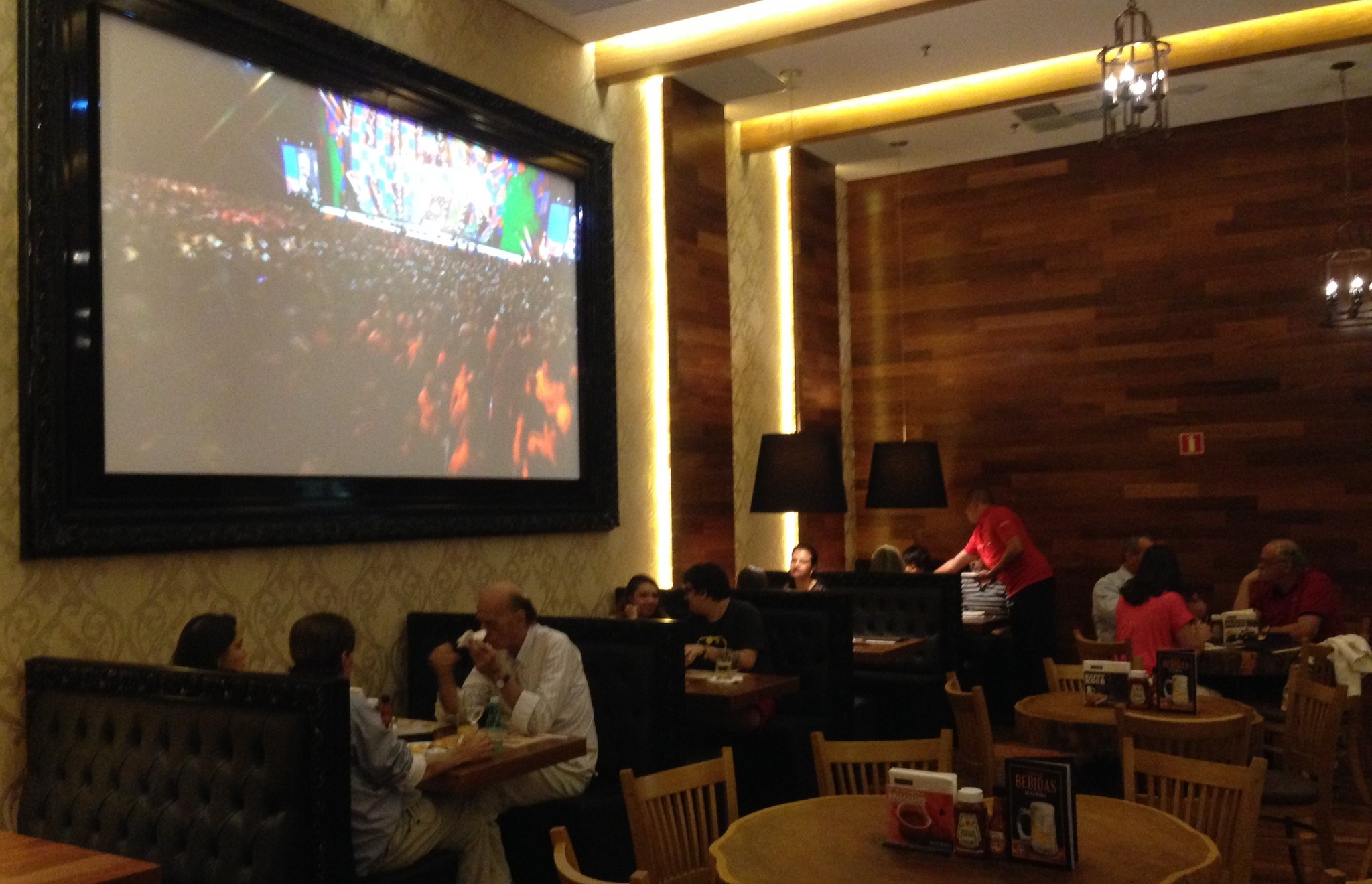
[545,595,1372,884]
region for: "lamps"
[862,439,950,512]
[1093,0,1179,149]
[1314,59,1371,323]
[743,432,850,520]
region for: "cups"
[1163,674,1189,705]
[897,801,934,840]
[1016,800,1059,857]
[714,649,734,681]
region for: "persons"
[900,542,933,574]
[166,609,250,670]
[1113,544,1213,685]
[928,487,1063,730]
[866,545,903,574]
[102,151,583,481]
[284,611,520,883]
[734,565,767,589]
[606,573,671,624]
[780,543,830,591]
[1223,538,1349,704]
[1089,533,1207,658]
[429,579,594,883]
[677,561,781,731]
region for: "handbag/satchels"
[1243,632,1302,654]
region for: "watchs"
[493,672,512,691]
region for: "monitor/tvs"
[16,0,620,558]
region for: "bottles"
[986,796,1007,856]
[1128,669,1153,711]
[954,786,991,859]
[486,696,504,756]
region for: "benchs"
[2,552,1007,880]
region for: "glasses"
[684,587,695,595]
[1260,557,1287,567]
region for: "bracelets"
[1242,577,1251,587]
[699,644,709,659]
